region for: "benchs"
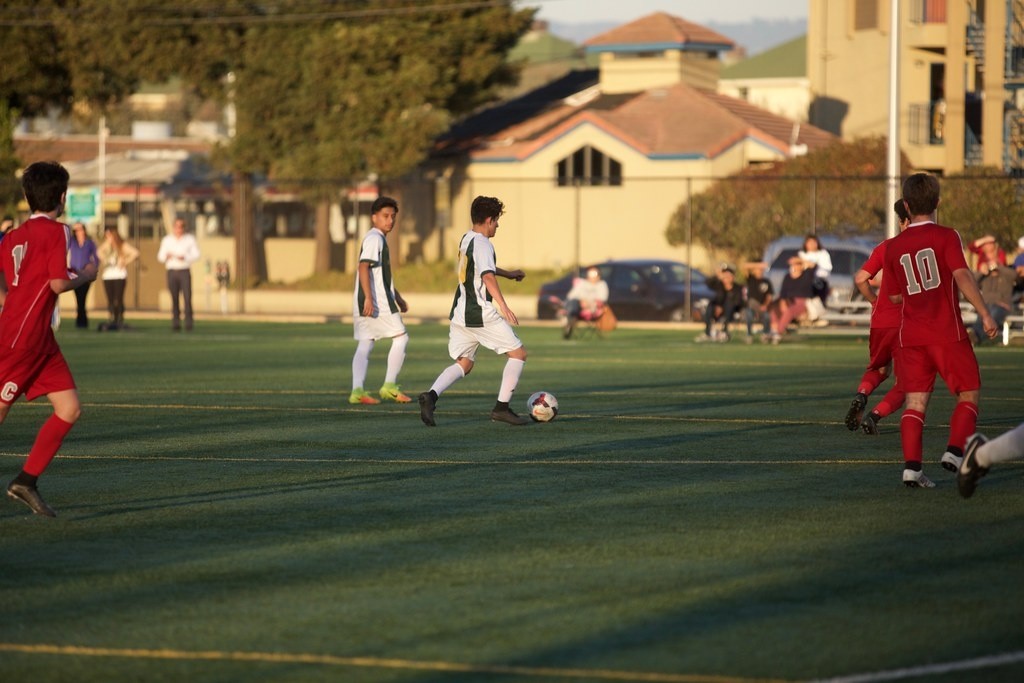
[731,301,1024,339]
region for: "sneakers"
[941,452,962,473]
[419,393,436,426]
[491,408,527,424]
[349,394,380,404]
[902,470,935,488]
[379,386,412,403]
[957,435,991,498]
[7,479,56,516]
[845,394,866,430]
[861,418,879,435]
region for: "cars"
[537,259,716,323]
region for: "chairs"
[571,276,605,340]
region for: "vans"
[762,236,893,316]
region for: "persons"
[957,423,1024,498]
[0,161,98,518]
[419,196,530,425]
[66,221,98,329]
[563,265,610,339]
[702,235,832,348]
[94,225,140,330]
[843,172,999,490]
[961,235,1024,347]
[156,218,199,331]
[348,196,413,404]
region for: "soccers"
[527,390,560,423]
